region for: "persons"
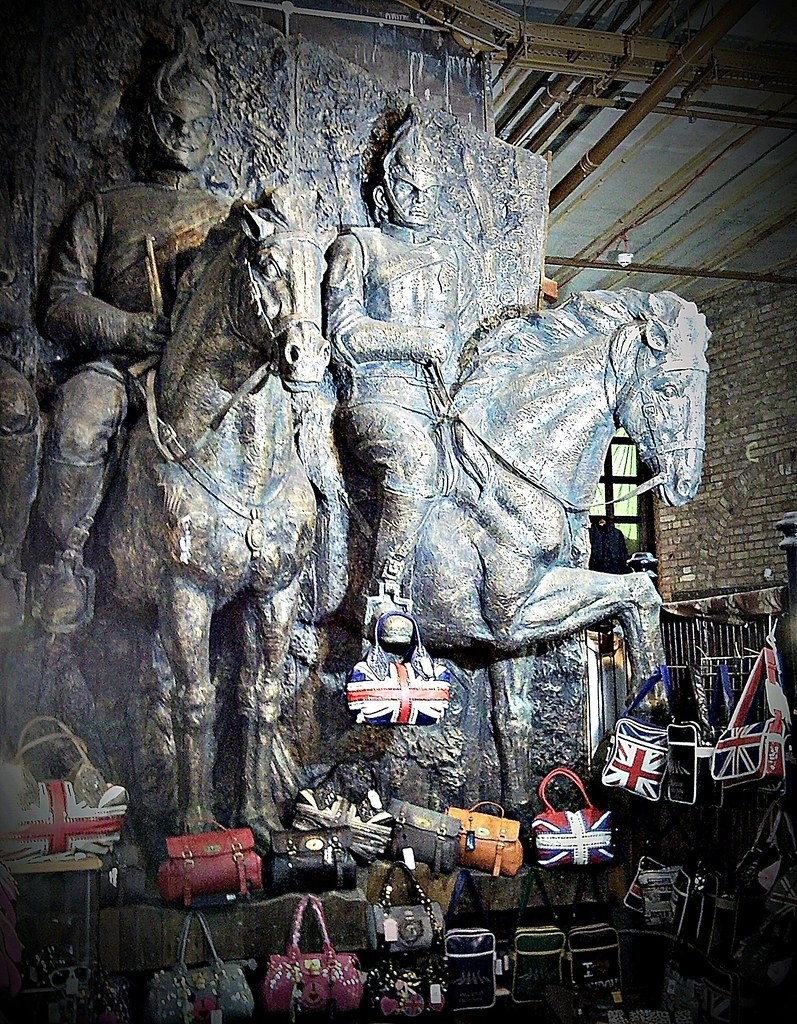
[28,17,259,631]
[325,119,476,643]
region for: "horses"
[100,195,713,848]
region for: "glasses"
[47,964,91,989]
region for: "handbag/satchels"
[293,758,396,865]
[0,715,129,864]
[157,818,263,908]
[444,802,523,878]
[146,910,255,1024]
[364,958,452,1023]
[528,767,618,868]
[340,611,451,726]
[366,861,446,959]
[379,793,463,880]
[504,868,568,1004]
[602,647,795,809]
[262,812,357,898]
[20,939,131,1023]
[624,799,796,1024]
[434,868,502,1012]
[553,866,623,1002]
[261,893,369,1023]
